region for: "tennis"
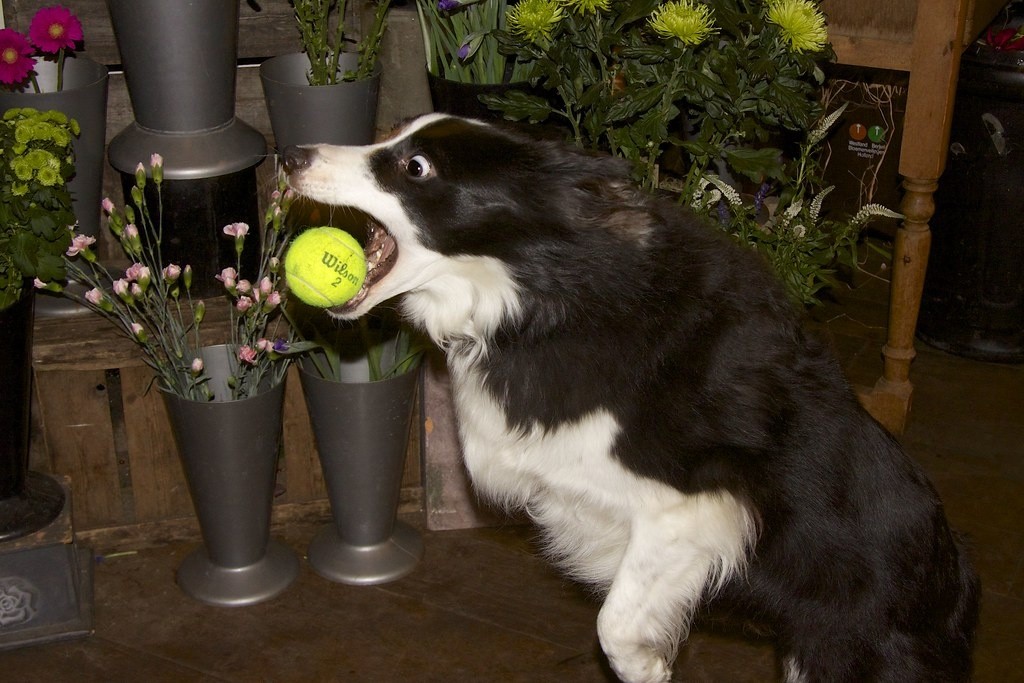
[285,225,369,309]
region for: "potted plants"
[259,0,391,158]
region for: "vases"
[426,53,549,125]
[1,294,64,542]
[296,330,422,587]
[103,0,268,180]
[0,56,128,319]
[156,344,296,607]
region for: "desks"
[810,0,1010,439]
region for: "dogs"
[274,112,983,683]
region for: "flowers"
[0,107,82,300]
[36,154,438,401]
[0,4,84,95]
[413,0,907,307]
[977,5,1024,53]
